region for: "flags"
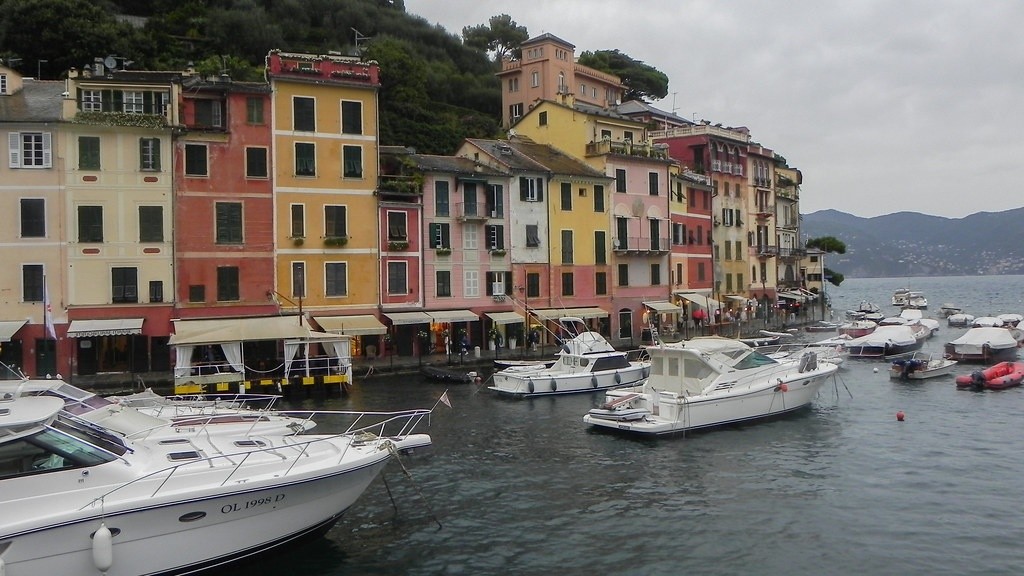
[46,278,58,340]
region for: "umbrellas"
[692,310,709,319]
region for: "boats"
[944,326,1024,362]
[888,352,958,380]
[956,362,1024,389]
[838,320,877,338]
[0,396,432,576]
[583,295,838,436]
[1,381,317,441]
[104,388,283,420]
[845,320,932,359]
[845,288,1024,328]
[739,320,841,347]
[487,291,651,396]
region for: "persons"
[444,334,452,355]
[459,332,469,356]
[725,308,752,328]
[529,329,540,352]
[779,305,799,324]
[694,318,700,332]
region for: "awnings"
[531,308,609,321]
[312,314,388,336]
[642,300,682,314]
[485,312,525,324]
[381,309,479,325]
[67,318,145,338]
[679,293,725,310]
[725,296,752,302]
[0,321,29,342]
